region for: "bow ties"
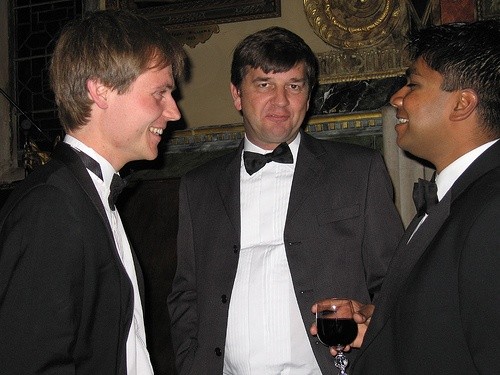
[78,150,136,211]
[243,141,294,176]
[413,177,439,217]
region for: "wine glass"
[316,295,359,375]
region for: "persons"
[166,25,409,375]
[0,10,185,375]
[311,18,500,373]
